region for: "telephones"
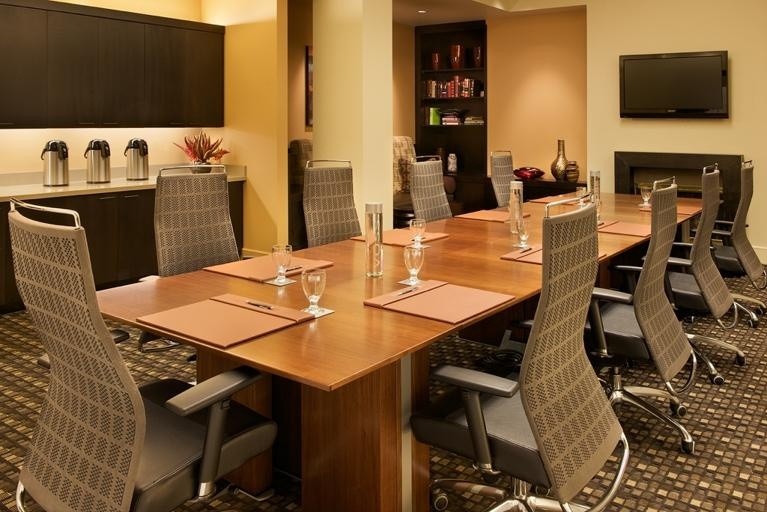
[513,167,544,179]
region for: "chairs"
[592,176,698,454]
[393,135,415,196]
[304,158,363,246]
[692,159,766,315]
[138,165,242,361]
[409,154,452,222]
[641,163,758,366]
[491,150,514,208]
[408,185,630,512]
[6,196,289,512]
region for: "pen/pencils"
[411,237,425,241]
[287,265,302,271]
[246,301,274,309]
[598,223,604,226]
[520,247,532,253]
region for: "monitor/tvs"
[619,51,728,118]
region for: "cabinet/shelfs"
[0,0,225,129]
[487,176,587,209]
[0,180,246,318]
[415,20,488,210]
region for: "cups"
[430,45,481,70]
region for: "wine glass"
[270,244,291,286]
[408,219,427,248]
[403,244,424,287]
[300,270,325,317]
[512,219,529,250]
[638,184,652,209]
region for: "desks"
[95,183,703,512]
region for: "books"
[423,74,485,125]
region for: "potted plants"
[175,134,229,174]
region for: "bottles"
[364,204,383,277]
[508,181,522,233]
[565,160,578,182]
[433,146,457,174]
[551,140,567,179]
[577,170,601,223]
